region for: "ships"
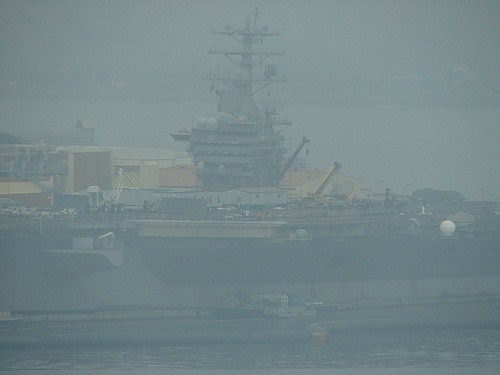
[3,4,499,347]
